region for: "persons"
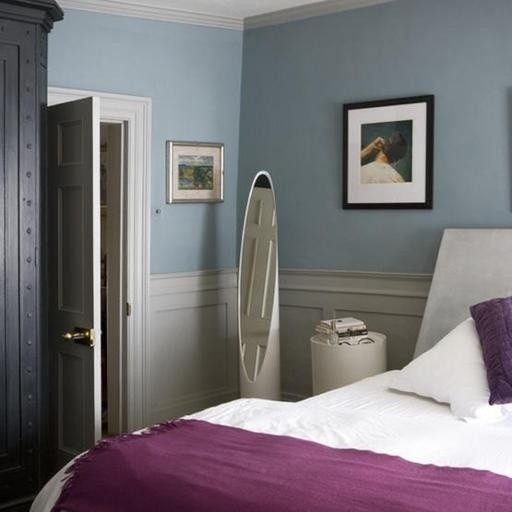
[360,123,413,185]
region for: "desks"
[310,330,387,396]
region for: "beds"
[34,226,512,512]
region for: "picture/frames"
[341,93,432,209]
[166,139,225,204]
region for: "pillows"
[470,294,512,408]
[385,317,508,422]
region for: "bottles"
[329,320,338,344]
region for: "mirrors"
[236,170,282,404]
